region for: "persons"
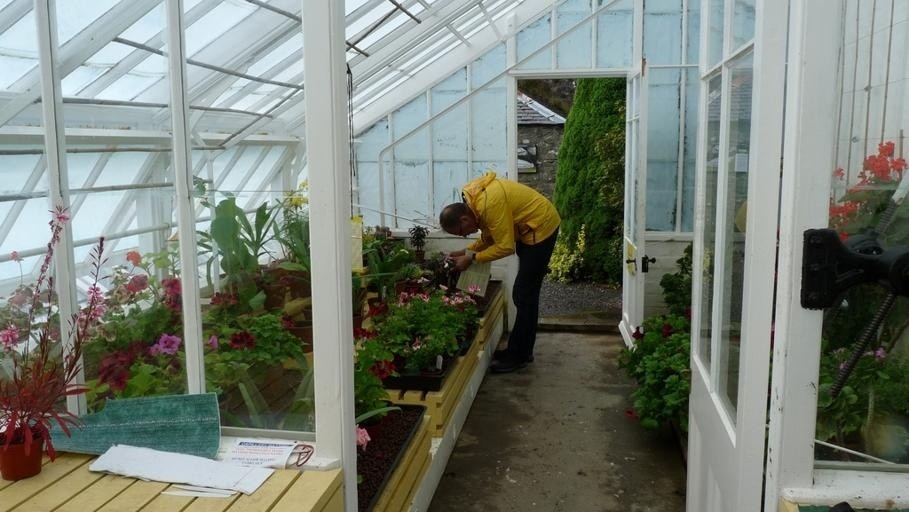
[440,172,560,373]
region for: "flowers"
[819,128,903,237]
[104,169,481,456]
[1,208,140,464]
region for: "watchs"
[471,253,477,265]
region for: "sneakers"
[489,346,535,374]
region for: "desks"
[2,451,346,512]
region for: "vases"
[1,428,46,481]
